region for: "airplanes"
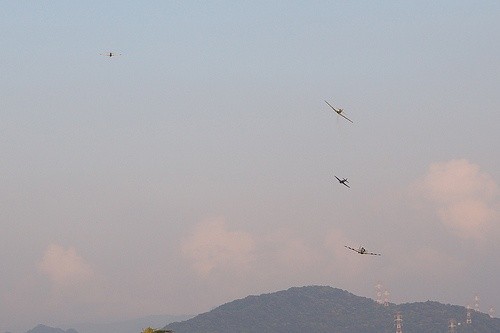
[345,245,381,256]
[324,100,354,124]
[335,176,350,189]
[99,51,122,57]
